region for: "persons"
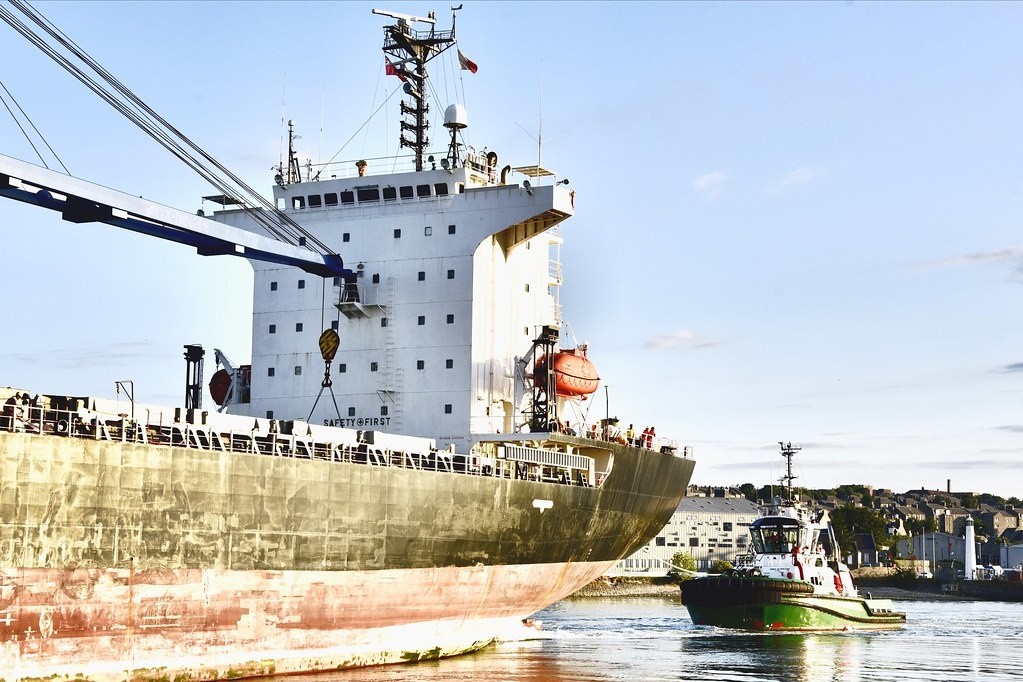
[641,425,656,450]
[3,392,25,420]
[624,424,635,446]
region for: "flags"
[458,49,478,75]
[385,55,408,82]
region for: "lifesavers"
[58,419,68,432]
[484,465,492,475]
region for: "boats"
[0,1,694,679]
[958,565,1023,601]
[678,506,907,638]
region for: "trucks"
[982,565,1003,578]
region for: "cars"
[916,571,933,580]
[862,562,871,567]
[872,562,884,567]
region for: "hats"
[16,392,23,398]
